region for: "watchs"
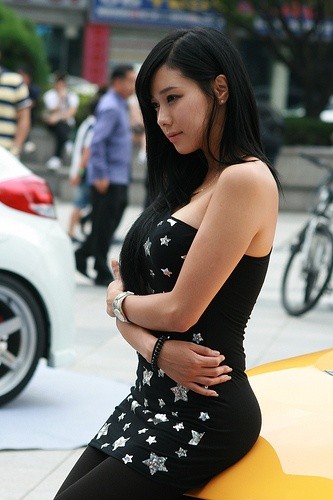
[112,290,135,324]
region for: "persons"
[0,58,147,284]
[52,28,284,500]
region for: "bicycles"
[279,152,333,318]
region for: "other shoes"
[24,140,35,152]
[48,156,61,168]
[78,217,87,237]
[70,235,82,244]
[94,276,114,286]
[65,141,73,153]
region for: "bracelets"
[151,336,166,371]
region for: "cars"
[0,145,77,407]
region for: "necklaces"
[194,168,222,195]
